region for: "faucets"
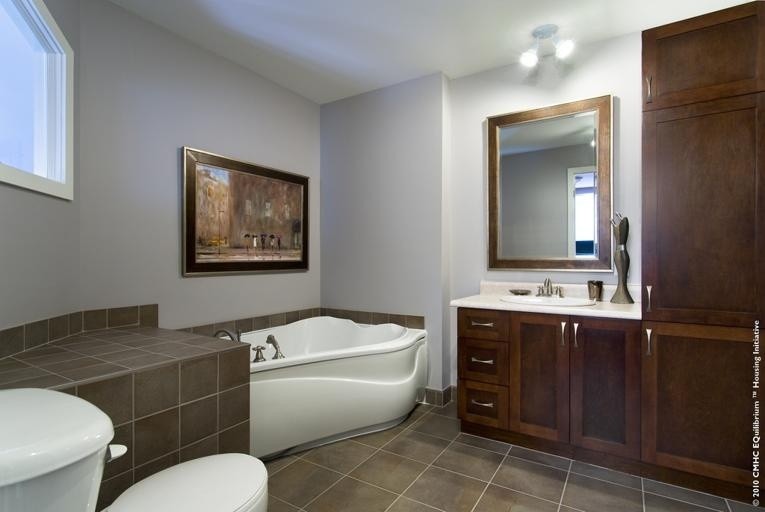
[543,276,553,296]
[266,334,285,360]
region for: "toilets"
[1,387,269,511]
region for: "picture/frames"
[179,146,311,277]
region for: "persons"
[245,235,281,251]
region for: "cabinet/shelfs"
[641,1,765,506]
[459,307,510,448]
[509,312,640,477]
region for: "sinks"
[499,296,596,307]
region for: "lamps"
[520,21,574,70]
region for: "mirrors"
[486,93,614,272]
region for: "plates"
[510,289,532,296]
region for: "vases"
[610,217,634,305]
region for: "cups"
[587,280,604,303]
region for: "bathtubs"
[218,315,428,463]
